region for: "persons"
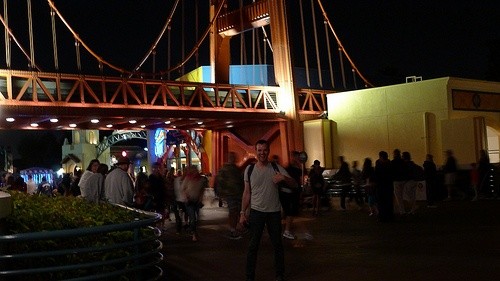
[239,139,299,281]
[0,156,209,242]
[213,146,492,240]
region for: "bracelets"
[239,207,246,214]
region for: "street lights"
[121,149,128,157]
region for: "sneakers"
[283,231,295,239]
[227,232,242,240]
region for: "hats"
[116,157,133,164]
